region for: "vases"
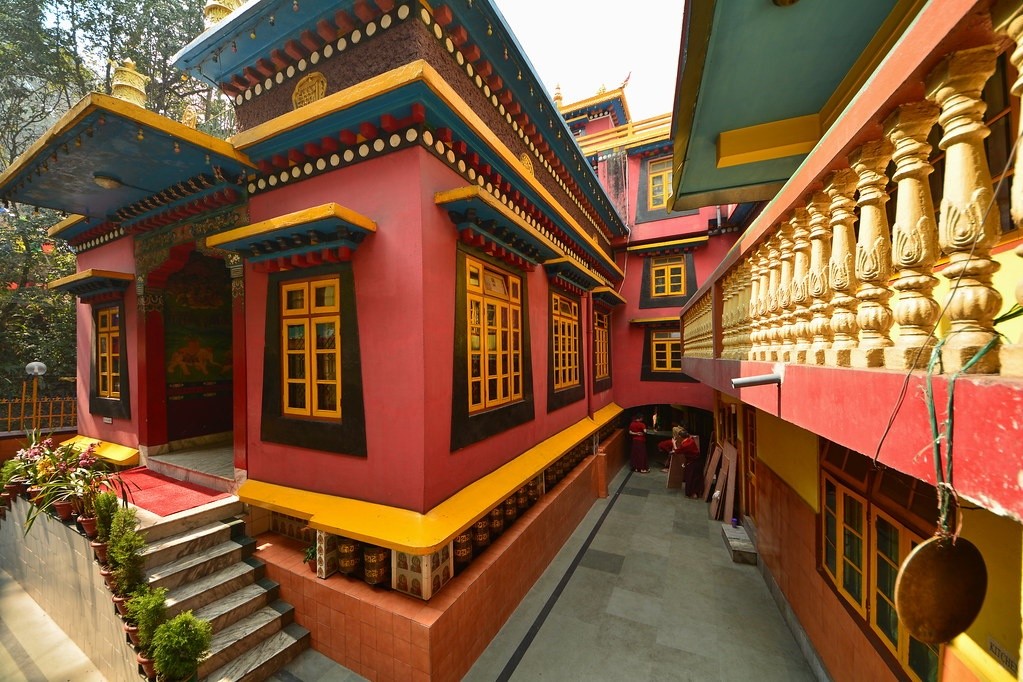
[71,512,78,525]
[77,512,95,537]
[21,482,32,499]
[27,486,46,502]
[50,500,73,518]
[12,476,28,494]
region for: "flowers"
[13,430,144,536]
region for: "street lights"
[25,362,47,431]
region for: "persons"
[629,414,650,473]
[672,429,702,499]
[657,440,674,471]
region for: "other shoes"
[641,469,650,472]
[689,495,697,499]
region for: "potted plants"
[3,460,21,495]
[96,494,211,682]
[302,548,317,574]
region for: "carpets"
[97,464,231,518]
